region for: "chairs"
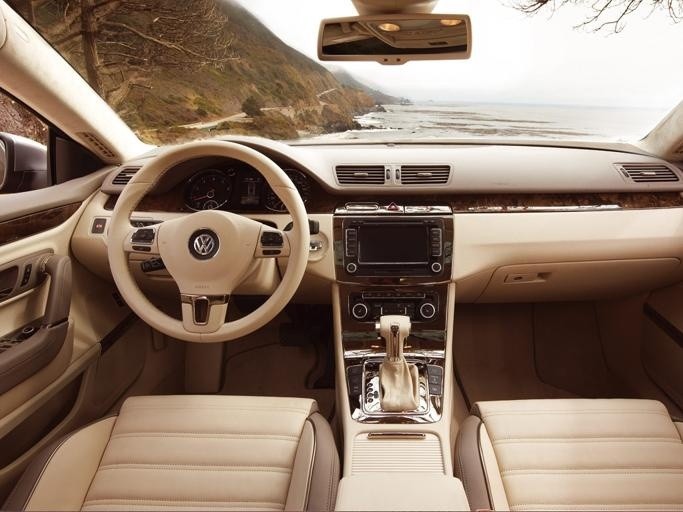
[3,393,341,510]
[455,392,682,510]
